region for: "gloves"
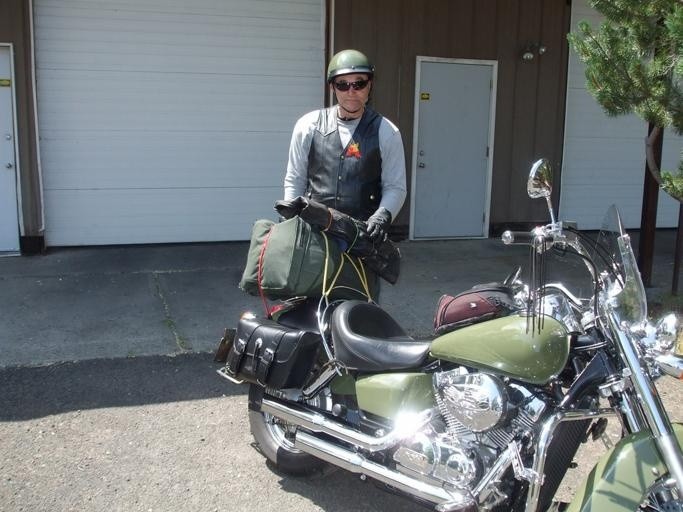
[365,207,393,244]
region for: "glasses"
[335,80,368,91]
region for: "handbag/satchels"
[239,215,381,305]
[433,282,515,335]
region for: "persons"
[283,48,408,306]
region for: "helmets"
[327,49,376,85]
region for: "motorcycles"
[212,157,683,512]
